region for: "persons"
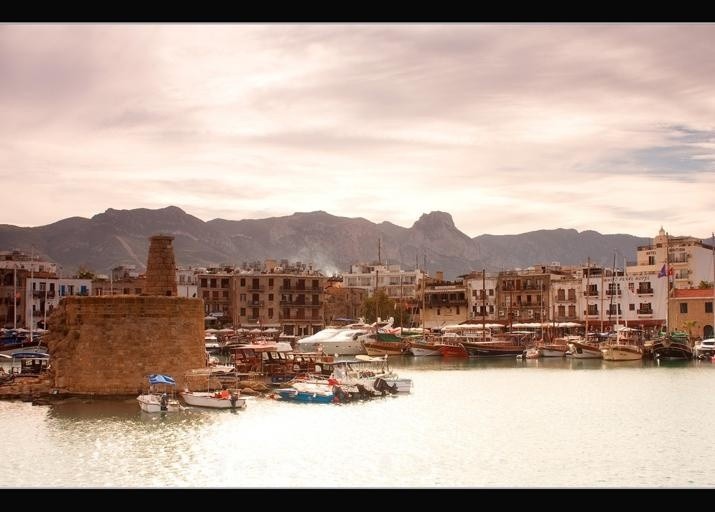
[220,384,229,399]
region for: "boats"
[0,352,49,377]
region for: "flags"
[669,267,674,276]
[657,263,667,278]
